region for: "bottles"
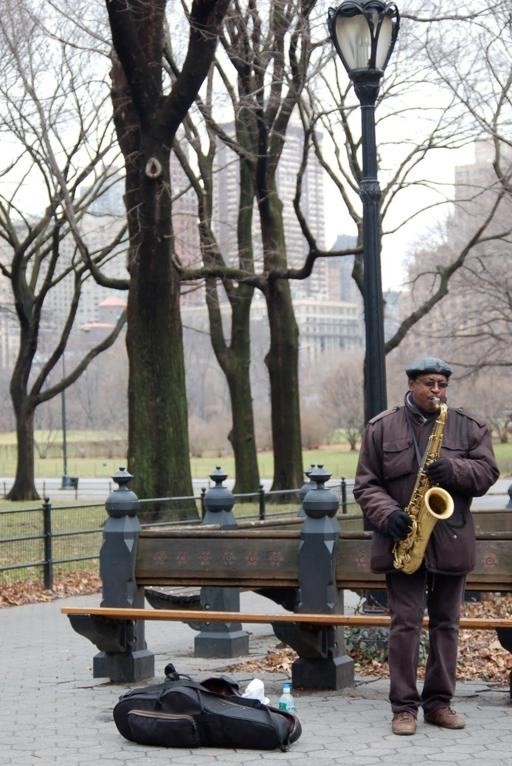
[279,681,296,718]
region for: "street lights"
[326,0,401,534]
[56,325,93,478]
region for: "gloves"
[388,511,412,539]
[426,458,451,483]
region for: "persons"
[349,356,500,738]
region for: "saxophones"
[392,396,454,575]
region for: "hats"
[406,357,452,378]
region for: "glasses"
[426,381,448,388]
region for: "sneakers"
[424,706,465,728]
[392,712,416,735]
